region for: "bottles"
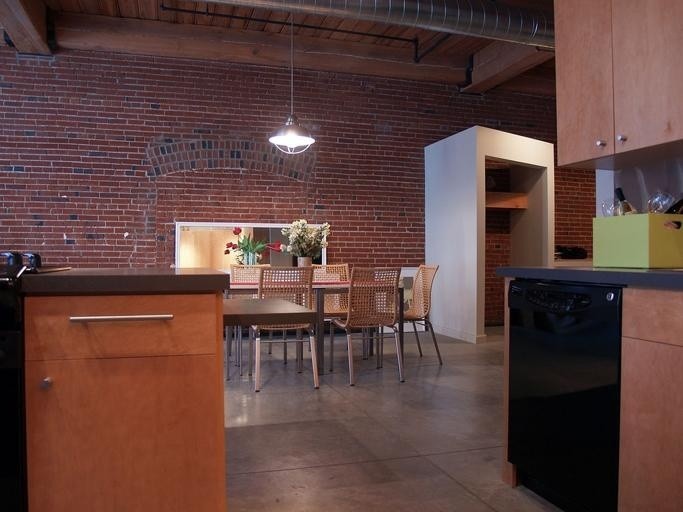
[614,187,638,214]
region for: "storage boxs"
[592,214,683,270]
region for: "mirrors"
[175,222,326,281]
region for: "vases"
[243,251,256,267]
[297,257,313,267]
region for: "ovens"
[505,280,618,512]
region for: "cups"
[646,191,674,213]
[602,201,624,216]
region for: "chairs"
[230,263,440,392]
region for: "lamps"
[268,13,315,155]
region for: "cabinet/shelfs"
[554,0,683,170]
[619,286,682,512]
[22,266,229,512]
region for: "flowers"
[280,219,331,257]
[223,226,281,261]
